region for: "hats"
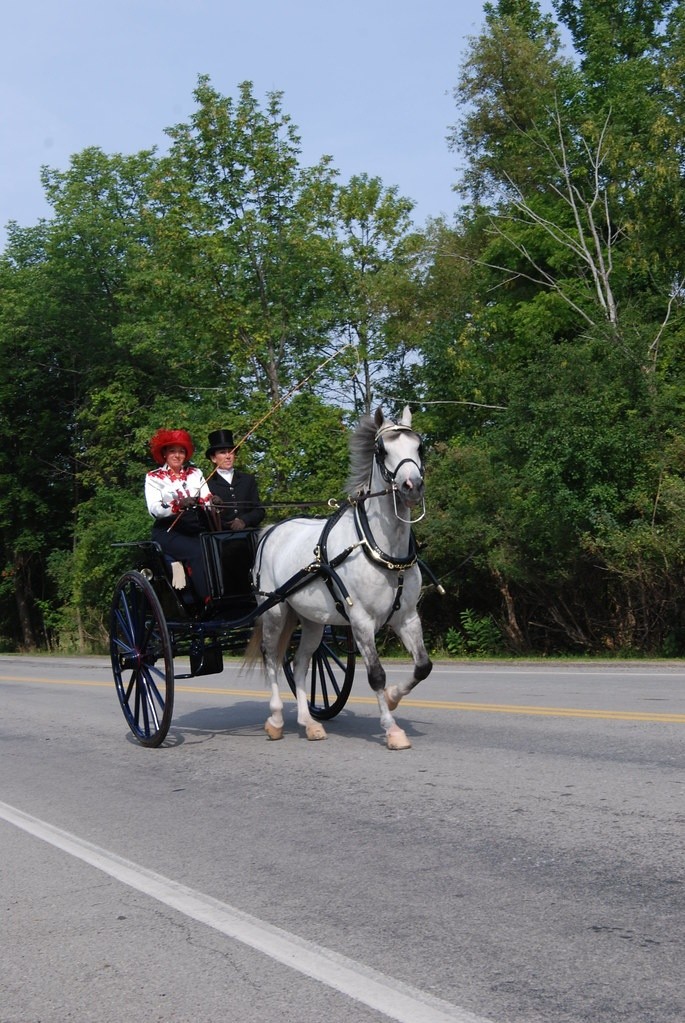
[206,430,240,460]
[149,428,195,466]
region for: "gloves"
[226,518,246,531]
[212,495,223,507]
[171,562,186,590]
[178,497,195,509]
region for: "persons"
[144,427,265,578]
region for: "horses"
[236,404,433,751]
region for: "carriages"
[104,405,434,752]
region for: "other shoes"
[204,595,221,609]
